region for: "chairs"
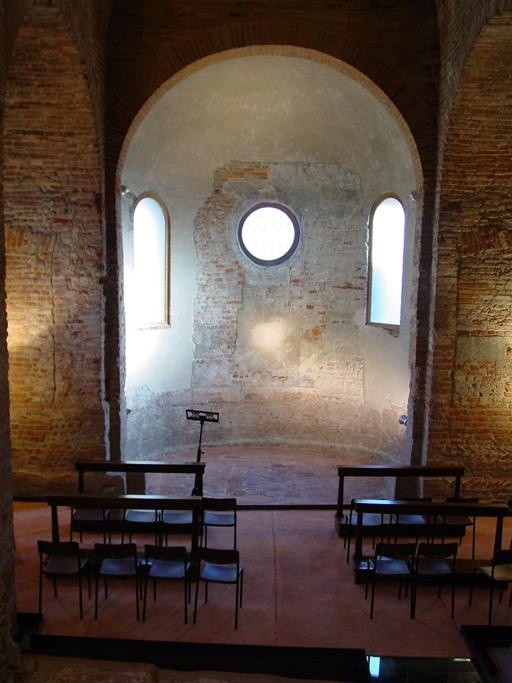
[30,458,249,632]
[337,463,512,625]
